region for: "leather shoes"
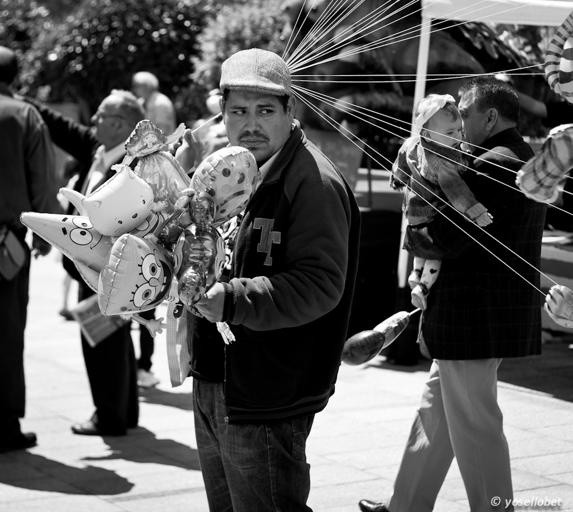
[70,419,122,434]
[358,496,388,511]
[0,429,36,452]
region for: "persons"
[131,70,177,138]
[183,47,363,511]
[1,46,62,454]
[389,93,495,311]
[19,120,257,318]
[134,325,161,388]
[14,88,144,437]
[357,74,549,511]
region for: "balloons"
[510,10,573,333]
[342,311,410,366]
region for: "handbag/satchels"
[0,226,23,281]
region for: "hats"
[413,92,458,133]
[218,46,295,97]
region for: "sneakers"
[409,283,427,312]
[138,365,159,388]
[408,269,421,290]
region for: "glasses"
[98,111,124,121]
